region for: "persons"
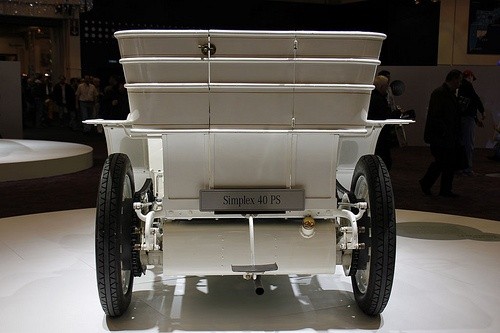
[17,70,128,141]
[369,69,406,173]
[415,67,485,201]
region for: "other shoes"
[439,191,459,199]
[418,179,433,196]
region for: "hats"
[462,70,478,81]
